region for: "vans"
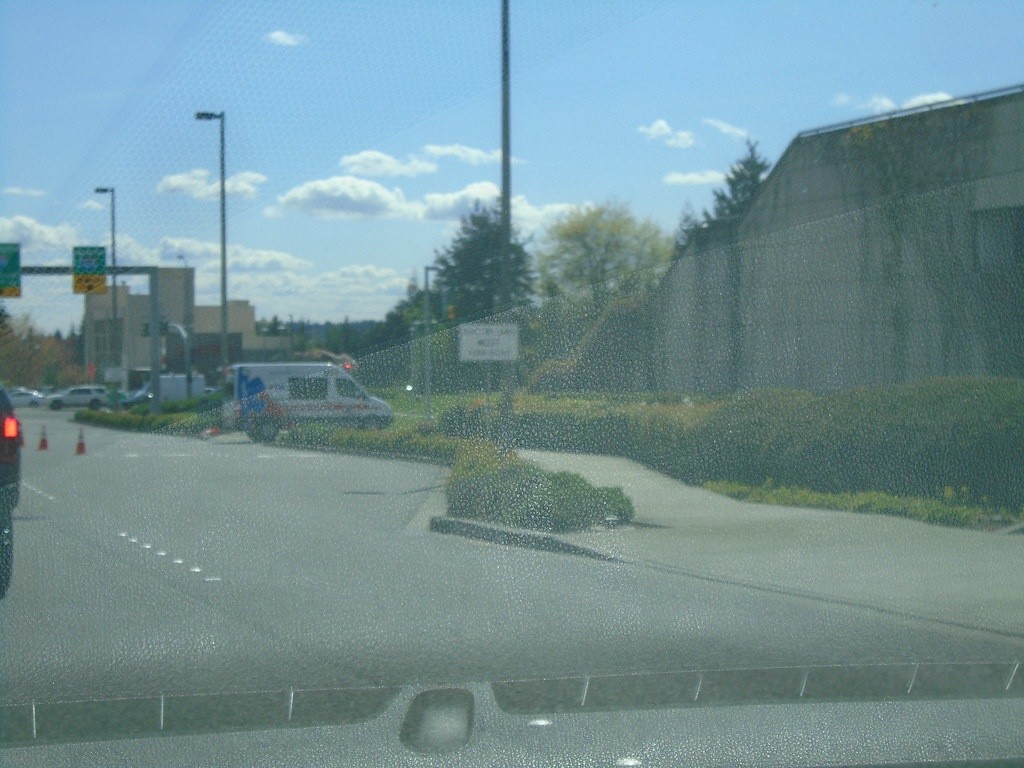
[233,361,397,444]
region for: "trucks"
[122,373,205,409]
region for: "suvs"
[44,386,109,411]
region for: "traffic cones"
[75,426,85,454]
[18,420,25,446]
[38,425,48,451]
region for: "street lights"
[92,186,122,366]
[194,109,229,379]
[422,262,446,422]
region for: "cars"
[7,389,46,410]
